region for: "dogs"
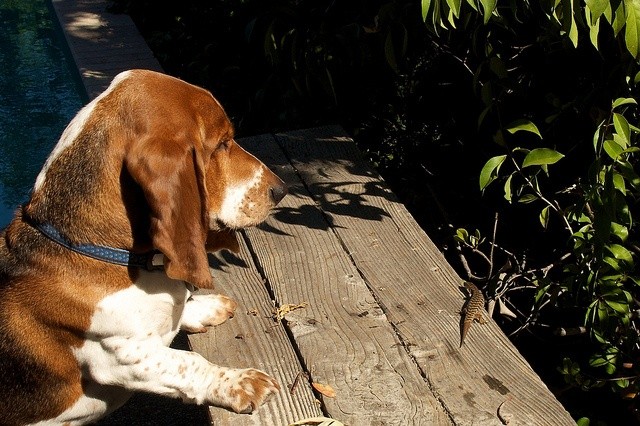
[1,68,290,426]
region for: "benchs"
[148,124,578,426]
